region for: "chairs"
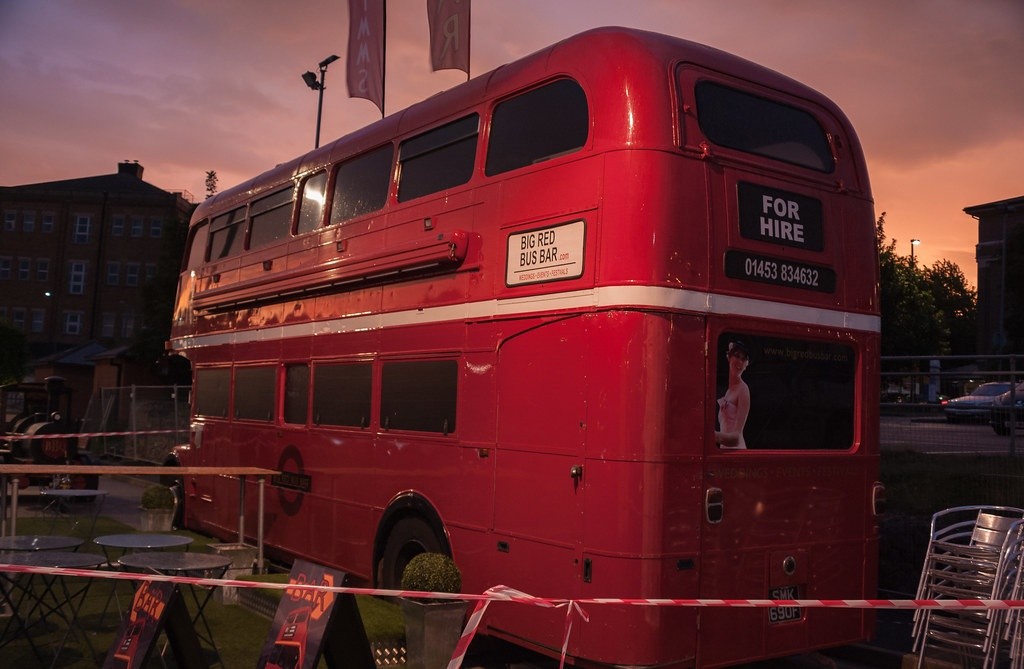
[911,505,1024,669]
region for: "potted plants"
[397,553,470,669]
[138,484,177,551]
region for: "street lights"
[911,239,920,262]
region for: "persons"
[716,338,753,449]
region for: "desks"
[0,533,233,669]
[0,463,282,617]
[39,489,109,551]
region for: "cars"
[940,381,1024,436]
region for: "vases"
[205,543,259,606]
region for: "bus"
[157,26,879,669]
